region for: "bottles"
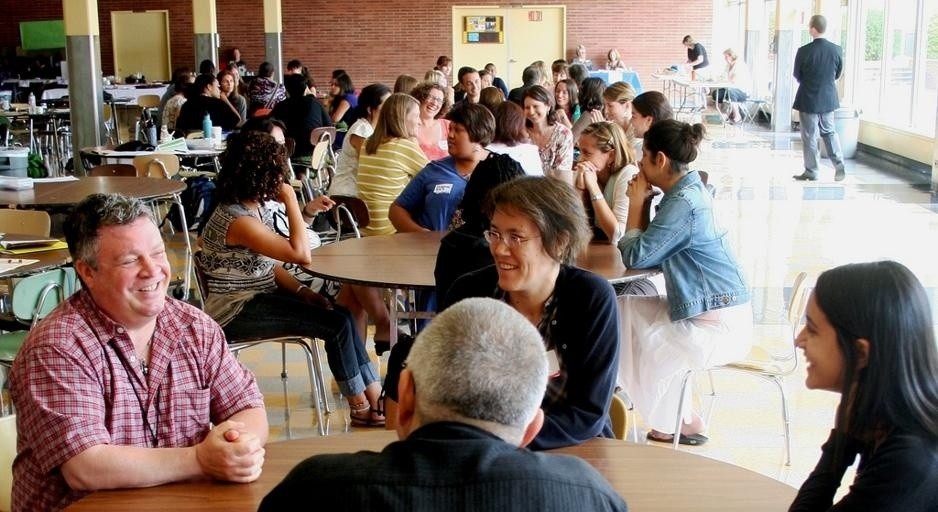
[28,93,37,115]
[202,113,212,140]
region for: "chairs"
[739,82,773,130]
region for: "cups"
[211,127,221,145]
[0,90,12,110]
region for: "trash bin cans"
[820,107,862,159]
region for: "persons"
[786,257,938,510]
[792,13,849,182]
[3,190,270,510]
[255,296,635,510]
[157,35,753,454]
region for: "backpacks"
[165,174,217,231]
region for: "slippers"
[647,430,707,445]
[373,334,413,356]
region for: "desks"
[589,70,637,88]
[651,72,734,129]
[1,78,170,106]
[63,429,803,512]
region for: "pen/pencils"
[174,149,187,153]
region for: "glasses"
[425,94,445,106]
[484,229,541,251]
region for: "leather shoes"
[793,174,817,181]
[835,170,845,180]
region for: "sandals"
[349,405,367,426]
[369,404,387,425]
